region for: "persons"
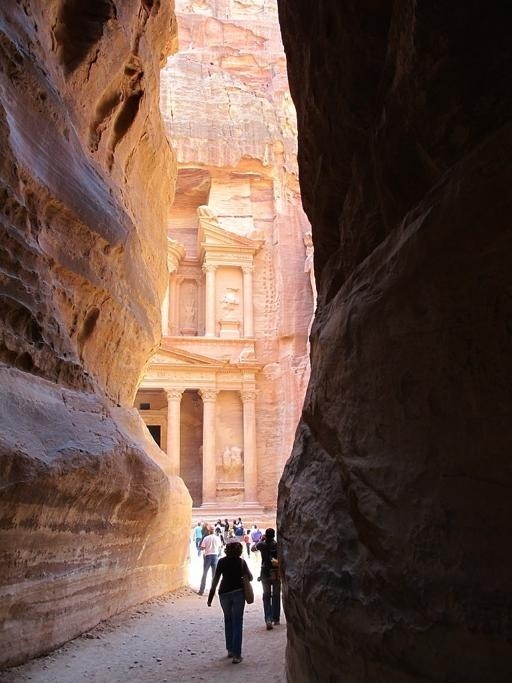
[251,527,282,630]
[197,523,223,596]
[192,515,263,559]
[207,541,254,665]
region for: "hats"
[263,528,275,538]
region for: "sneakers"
[275,619,279,625]
[233,656,242,663]
[228,651,232,656]
[267,622,273,629]
[197,592,202,595]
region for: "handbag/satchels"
[241,575,254,604]
